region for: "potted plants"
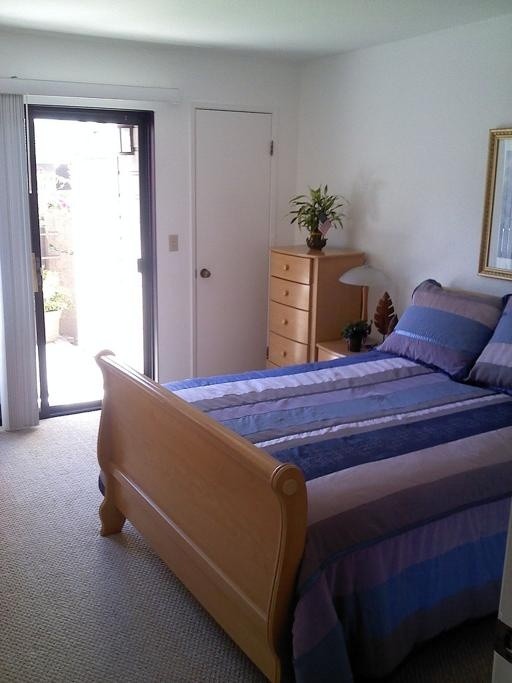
[41,264,74,343]
[341,320,371,353]
[282,184,349,251]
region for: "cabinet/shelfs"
[265,247,368,369]
[40,233,61,259]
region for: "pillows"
[376,278,508,382]
[463,296,510,396]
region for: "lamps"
[339,264,389,346]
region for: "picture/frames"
[120,126,134,155]
[479,129,511,279]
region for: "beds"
[95,279,511,682]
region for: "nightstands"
[315,338,383,362]
